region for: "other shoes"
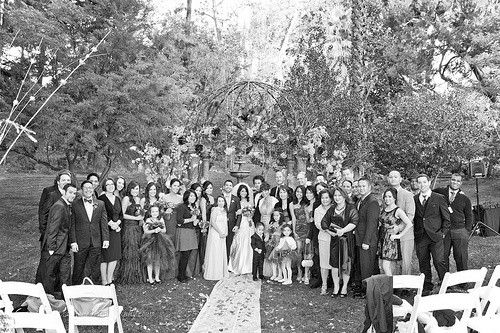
[106,275,471,299]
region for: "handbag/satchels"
[66,276,114,317]
[329,223,342,232]
[300,259,314,267]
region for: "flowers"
[135,206,145,226]
[241,205,255,227]
[151,221,164,229]
[190,209,200,226]
[198,220,210,236]
[266,225,277,240]
[164,201,175,209]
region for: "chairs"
[361,264,500,333]
[0,279,66,333]
[62,283,124,333]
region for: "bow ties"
[84,198,93,204]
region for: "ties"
[449,192,456,204]
[422,195,428,207]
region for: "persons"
[140,183,165,232]
[406,174,421,196]
[143,203,178,285]
[39,168,72,224]
[412,174,453,295]
[100,175,127,204]
[34,171,73,287]
[430,172,473,289]
[162,165,380,300]
[378,188,414,295]
[381,170,416,296]
[39,184,77,301]
[98,177,124,287]
[120,180,147,284]
[77,173,99,202]
[70,179,110,285]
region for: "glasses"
[106,184,114,186]
